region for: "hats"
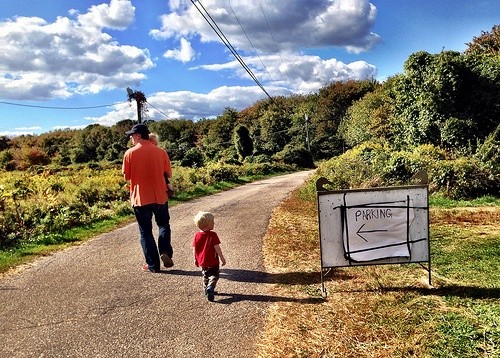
[125,124,151,134]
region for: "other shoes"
[142,264,160,273]
[168,189,174,195]
[207,286,214,301]
[160,254,174,268]
[205,288,207,295]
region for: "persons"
[193,211,227,302]
[125,134,174,195]
[122,124,174,272]
